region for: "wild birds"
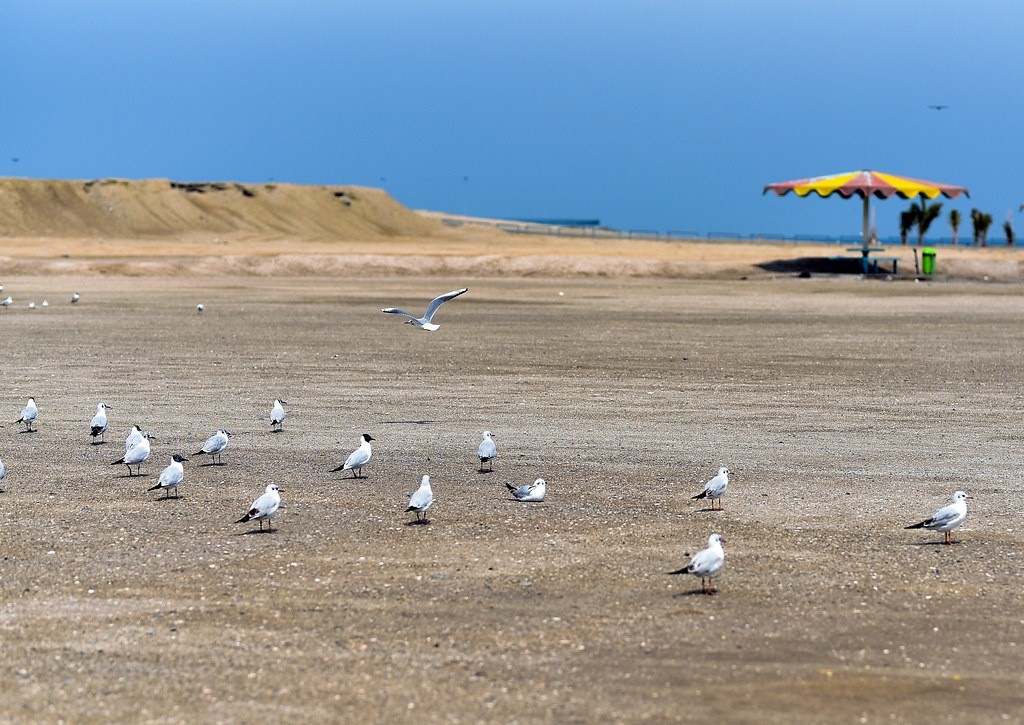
[192,428,231,464]
[269,399,287,433]
[691,468,734,510]
[147,454,189,498]
[197,304,204,313]
[110,424,157,477]
[70,293,79,304]
[89,403,112,444]
[234,484,285,532]
[15,397,38,433]
[29,300,48,309]
[477,431,497,471]
[328,433,376,479]
[506,477,548,502]
[380,288,468,331]
[929,105,946,110]
[404,474,434,522]
[904,490,974,545]
[0,296,12,307]
[666,533,727,595]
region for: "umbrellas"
[763,169,969,248]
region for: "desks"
[846,248,884,273]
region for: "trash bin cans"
[922,248,936,274]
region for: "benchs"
[860,257,900,274]
